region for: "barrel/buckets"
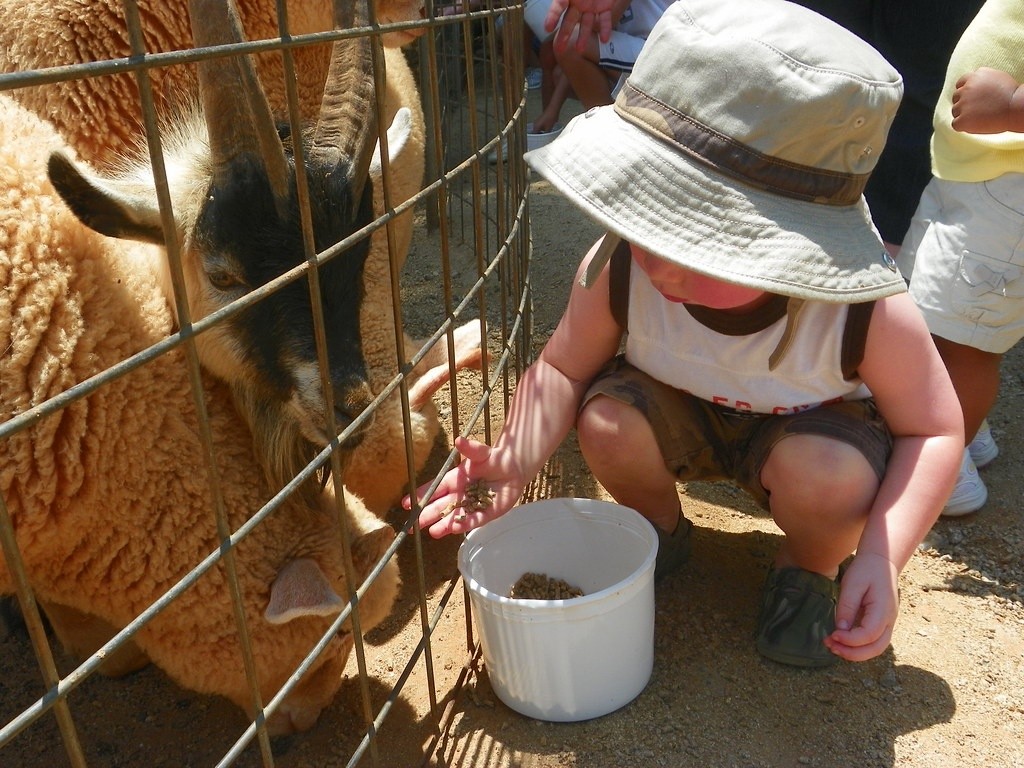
[457,497,660,722]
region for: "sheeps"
[0,1,495,736]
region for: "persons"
[430,0,1024,516]
[403,0,965,668]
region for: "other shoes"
[754,556,844,669]
[521,65,542,90]
[646,502,695,582]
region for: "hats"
[519,0,911,305]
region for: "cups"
[527,122,564,171]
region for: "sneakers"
[967,418,999,467]
[939,445,988,518]
